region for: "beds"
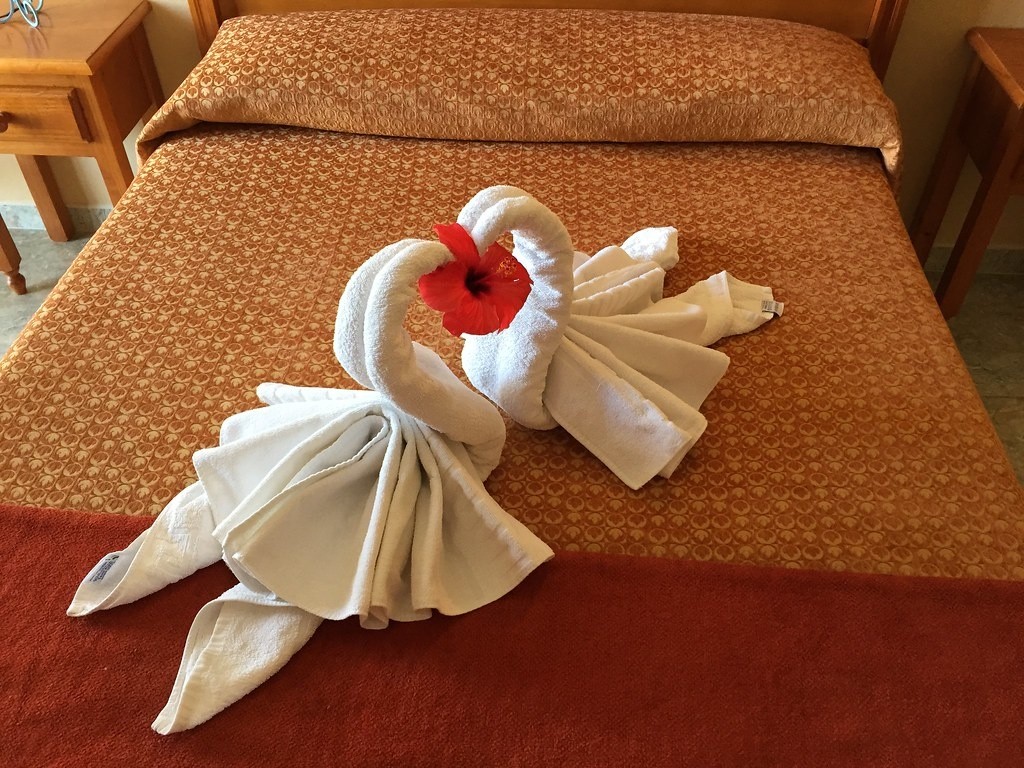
[0,0,1024,768]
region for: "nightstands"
[0,0,166,298]
[906,25,1024,323]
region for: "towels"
[61,183,787,736]
